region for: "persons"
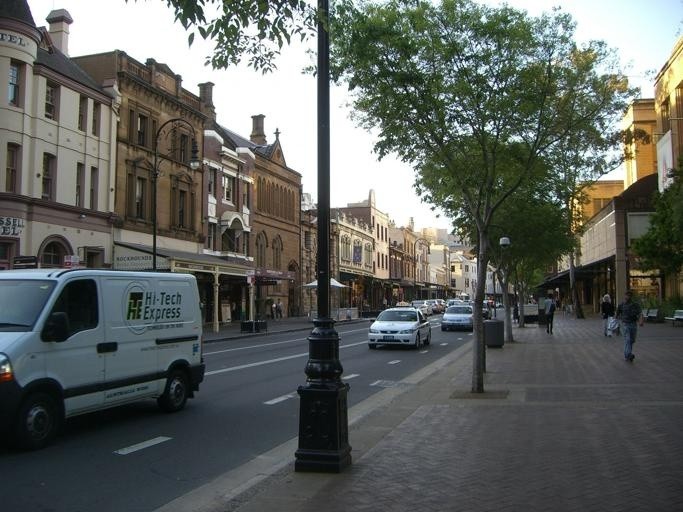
[543,294,554,334]
[614,290,643,361]
[600,292,616,337]
[275,298,283,317]
[511,297,565,324]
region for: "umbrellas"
[302,278,349,289]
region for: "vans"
[0,265,209,450]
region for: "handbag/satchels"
[548,304,555,313]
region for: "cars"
[409,297,474,315]
[441,305,473,331]
[367,307,432,351]
[471,300,493,320]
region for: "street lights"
[476,224,512,374]
[153,117,201,270]
[412,238,431,299]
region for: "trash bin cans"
[241,321,267,333]
[483,319,504,348]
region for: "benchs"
[641,307,661,326]
[663,309,682,328]
